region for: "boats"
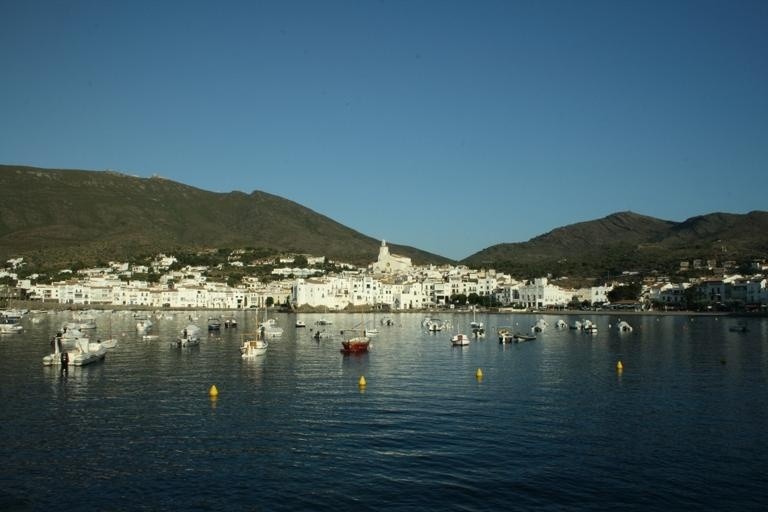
[730,320,750,331]
